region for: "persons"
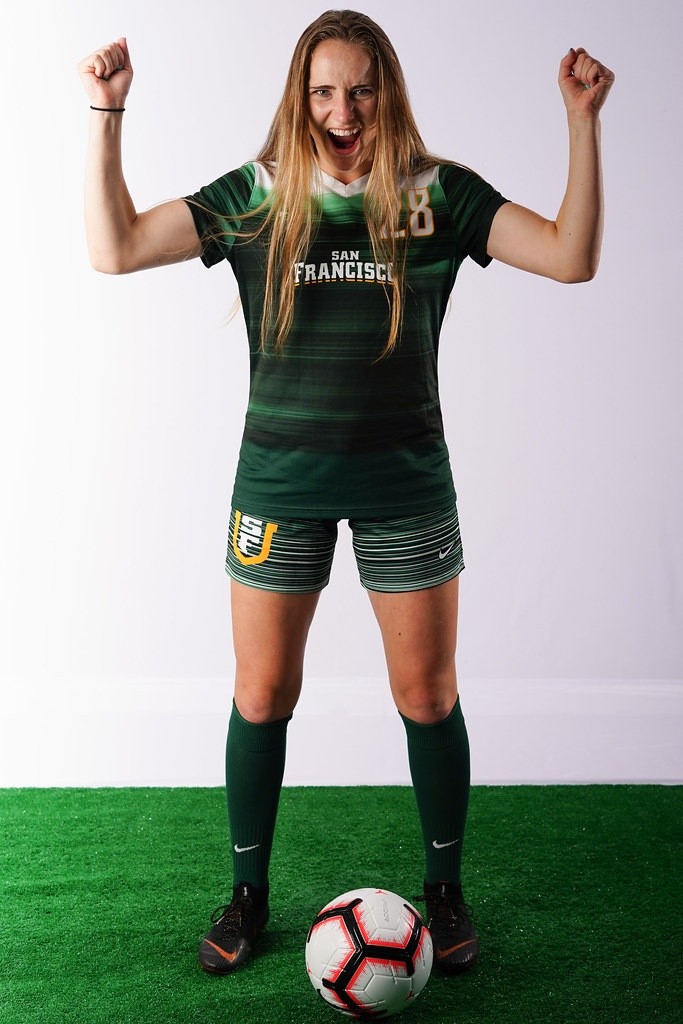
[83,9,612,970]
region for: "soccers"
[303,887,434,1021]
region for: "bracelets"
[90,106,125,112]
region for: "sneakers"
[198,880,271,975]
[421,879,478,972]
